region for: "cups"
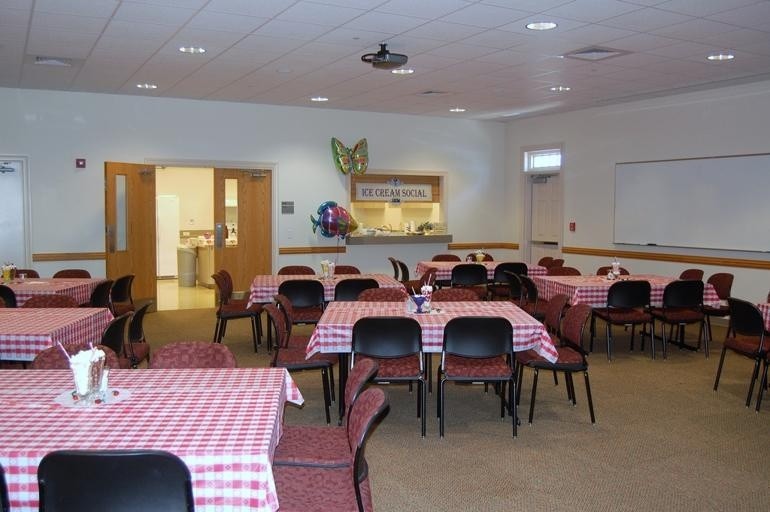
[319,257,336,280]
[19,273,27,281]
[421,286,432,309]
[404,298,414,314]
[466,256,473,264]
[473,248,487,265]
[2,263,16,284]
[68,354,109,408]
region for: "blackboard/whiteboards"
[612,153,770,252]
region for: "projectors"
[361,51,408,70]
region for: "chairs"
[270,358,381,469]
[35,447,200,511]
[334,265,362,275]
[596,264,631,275]
[538,254,582,275]
[488,261,549,319]
[278,278,327,330]
[515,293,598,426]
[641,279,710,362]
[438,313,519,440]
[432,254,462,289]
[467,254,494,262]
[588,278,656,364]
[271,384,391,511]
[711,290,770,417]
[210,269,263,354]
[335,278,379,303]
[1,254,153,370]
[263,292,339,427]
[388,257,410,283]
[703,271,736,343]
[147,340,239,368]
[277,266,316,275]
[668,268,704,341]
[451,263,490,291]
[348,316,431,440]
[430,289,480,301]
[358,287,411,302]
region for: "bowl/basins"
[366,229,376,237]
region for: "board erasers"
[647,244,656,245]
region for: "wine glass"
[412,294,426,313]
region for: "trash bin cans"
[177,245,198,286]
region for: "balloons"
[310,201,358,238]
[331,137,369,176]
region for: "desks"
[247,272,419,357]
[0,364,306,511]
[307,300,562,426]
[417,260,551,288]
[532,274,720,364]
